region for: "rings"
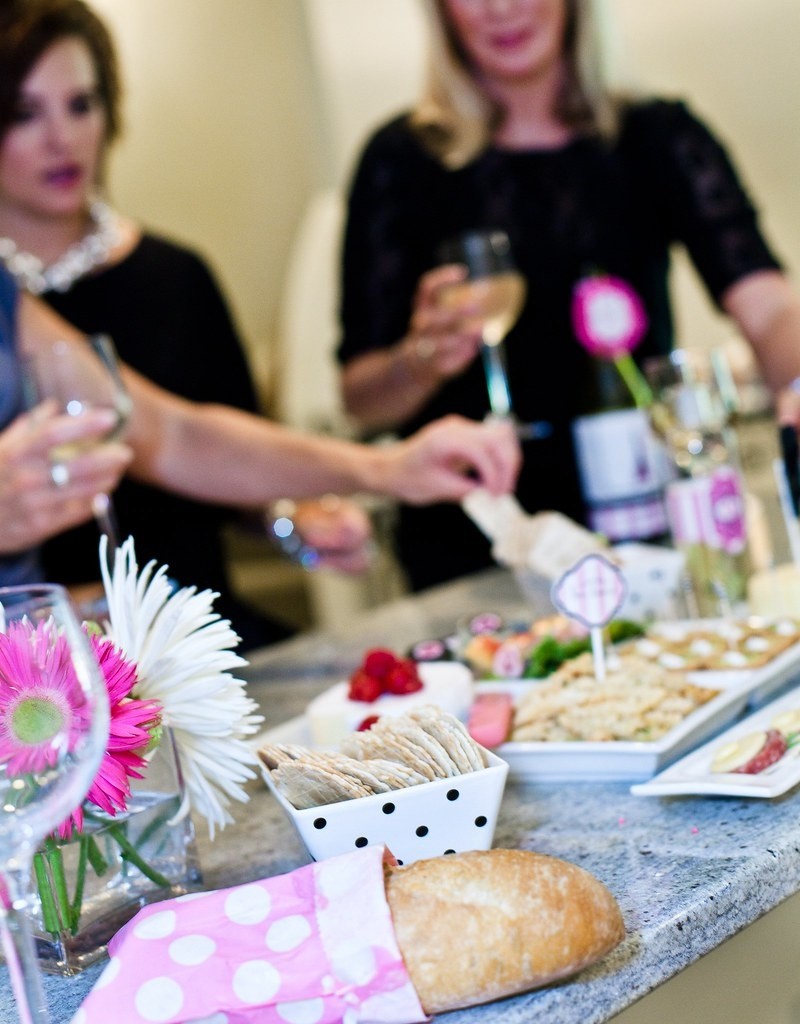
[50,463,68,487]
[417,340,435,357]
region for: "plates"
[631,685,800,797]
[247,637,800,780]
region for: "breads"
[381,848,627,1012]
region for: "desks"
[193,571,800,1021]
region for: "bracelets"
[271,498,318,566]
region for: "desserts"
[306,649,477,748]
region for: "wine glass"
[18,337,132,556]
[1,585,110,1023]
[446,233,522,417]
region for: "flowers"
[0,530,265,934]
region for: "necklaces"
[0,196,122,294]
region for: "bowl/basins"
[261,743,509,862]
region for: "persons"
[0,0,370,655]
[335,0,799,592]
[0,265,521,607]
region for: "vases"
[0,726,204,977]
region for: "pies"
[256,706,491,811]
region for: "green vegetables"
[478,618,647,680]
